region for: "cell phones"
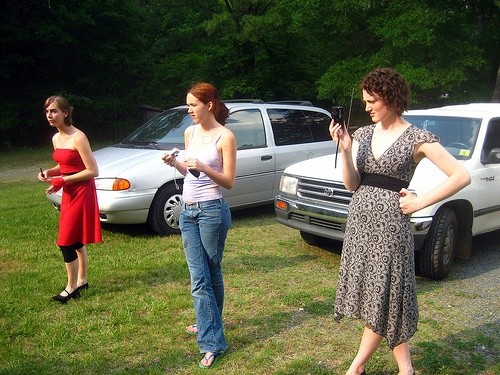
[40,168,44,177]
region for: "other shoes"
[52,288,81,303]
[77,282,88,291]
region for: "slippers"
[199,352,223,369]
[185,324,198,335]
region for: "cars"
[46,99,341,237]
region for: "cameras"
[167,148,180,158]
[331,106,343,129]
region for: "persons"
[329,68,471,375]
[38,96,99,303]
[162,83,237,370]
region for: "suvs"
[274,102,500,281]
